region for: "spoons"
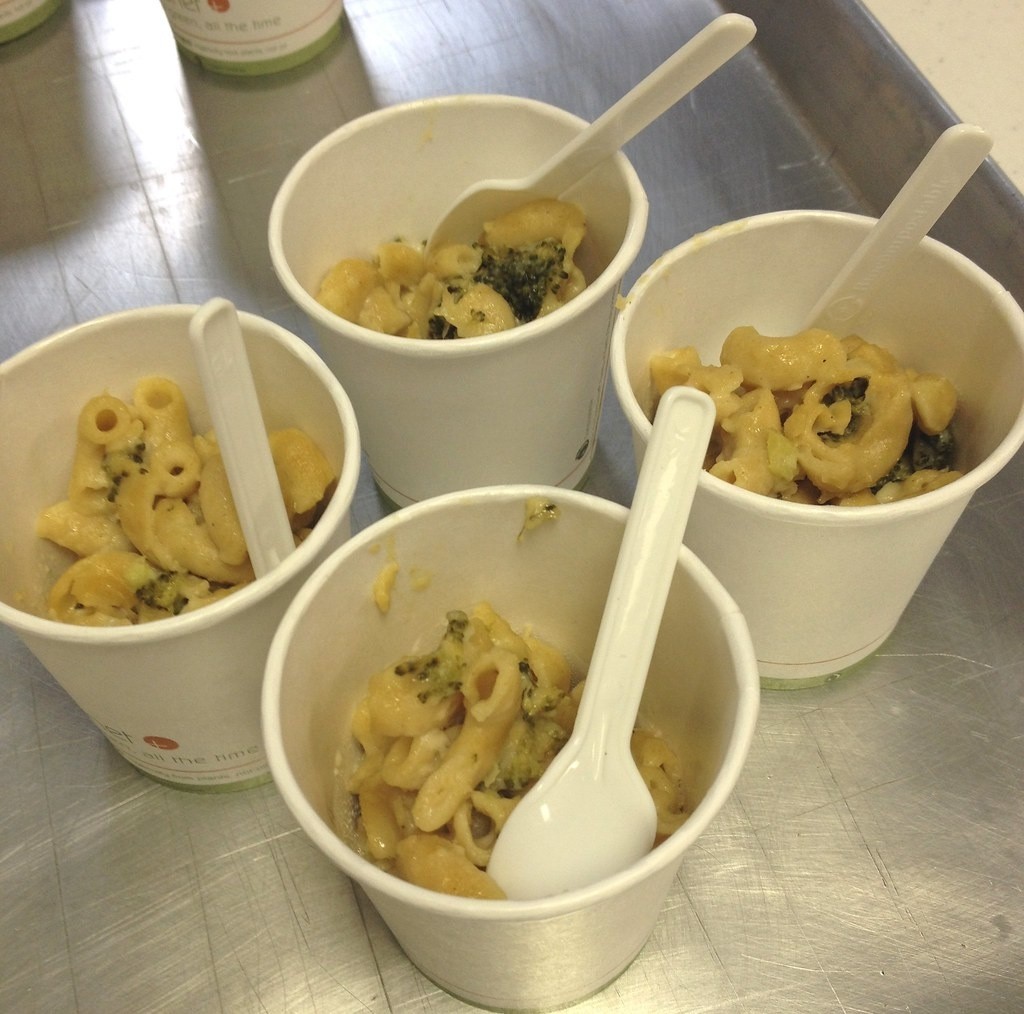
[489,386,716,907]
[426,14,758,262]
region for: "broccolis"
[822,378,950,481]
[484,240,569,323]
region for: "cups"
[269,94,650,504]
[0,0,63,45]
[611,209,1024,680]
[262,486,757,1012]
[159,0,345,76]
[0,303,362,786]
